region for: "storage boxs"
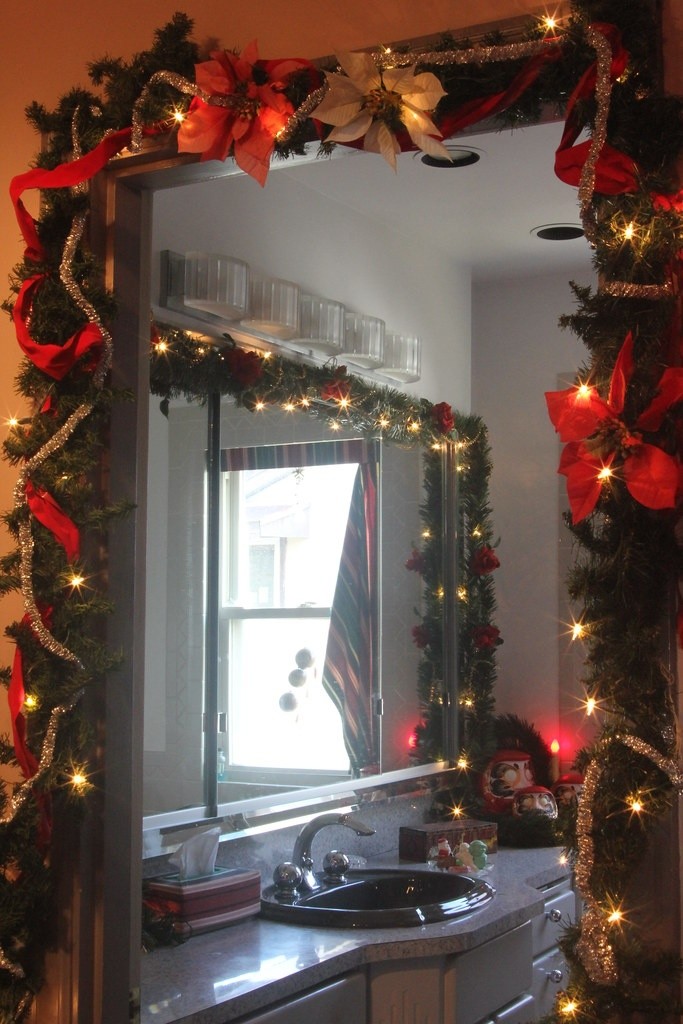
[399,819,498,864]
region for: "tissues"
[142,826,262,936]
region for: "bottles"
[216,747,226,782]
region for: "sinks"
[257,866,499,928]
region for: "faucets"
[291,812,377,897]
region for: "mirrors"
[142,321,460,849]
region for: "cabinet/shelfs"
[369,955,444,1024]
[532,879,576,1021]
[244,973,367,1024]
[445,920,532,1024]
